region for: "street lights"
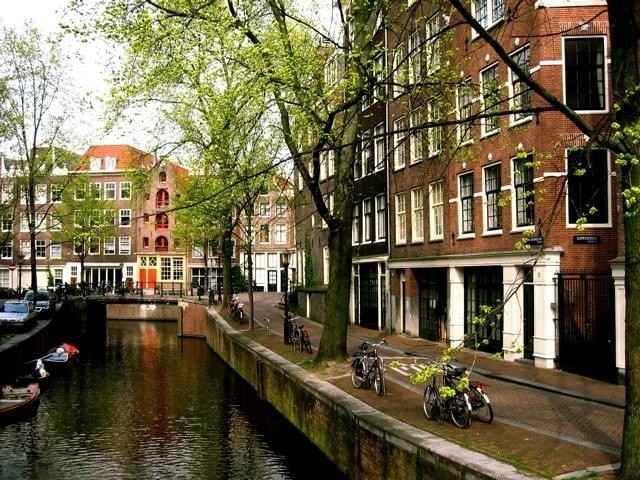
[16,254,25,297]
[281,248,296,345]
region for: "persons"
[5,286,26,299]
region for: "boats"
[16,344,78,383]
[0,382,42,415]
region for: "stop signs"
[9,263,16,272]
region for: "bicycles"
[282,313,298,344]
[420,362,472,429]
[55,281,129,299]
[290,314,313,356]
[235,297,245,325]
[0,322,14,340]
[443,363,494,426]
[228,295,237,321]
[349,336,389,394]
[0,287,30,299]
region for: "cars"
[0,298,36,331]
[23,290,55,317]
